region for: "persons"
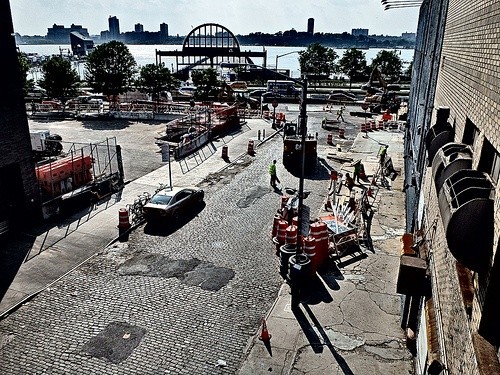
[344,173,354,192]
[380,145,389,164]
[353,159,362,183]
[269,160,277,186]
[337,107,344,122]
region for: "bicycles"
[125,182,169,222]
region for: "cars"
[142,186,205,222]
[362,82,410,103]
[329,93,356,101]
[248,89,266,96]
[330,89,356,97]
[261,92,284,98]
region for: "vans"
[230,81,247,88]
[41,98,63,107]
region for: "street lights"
[220,60,308,312]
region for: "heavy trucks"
[31,129,63,156]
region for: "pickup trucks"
[64,95,105,108]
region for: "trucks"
[266,79,301,95]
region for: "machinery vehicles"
[282,122,318,167]
